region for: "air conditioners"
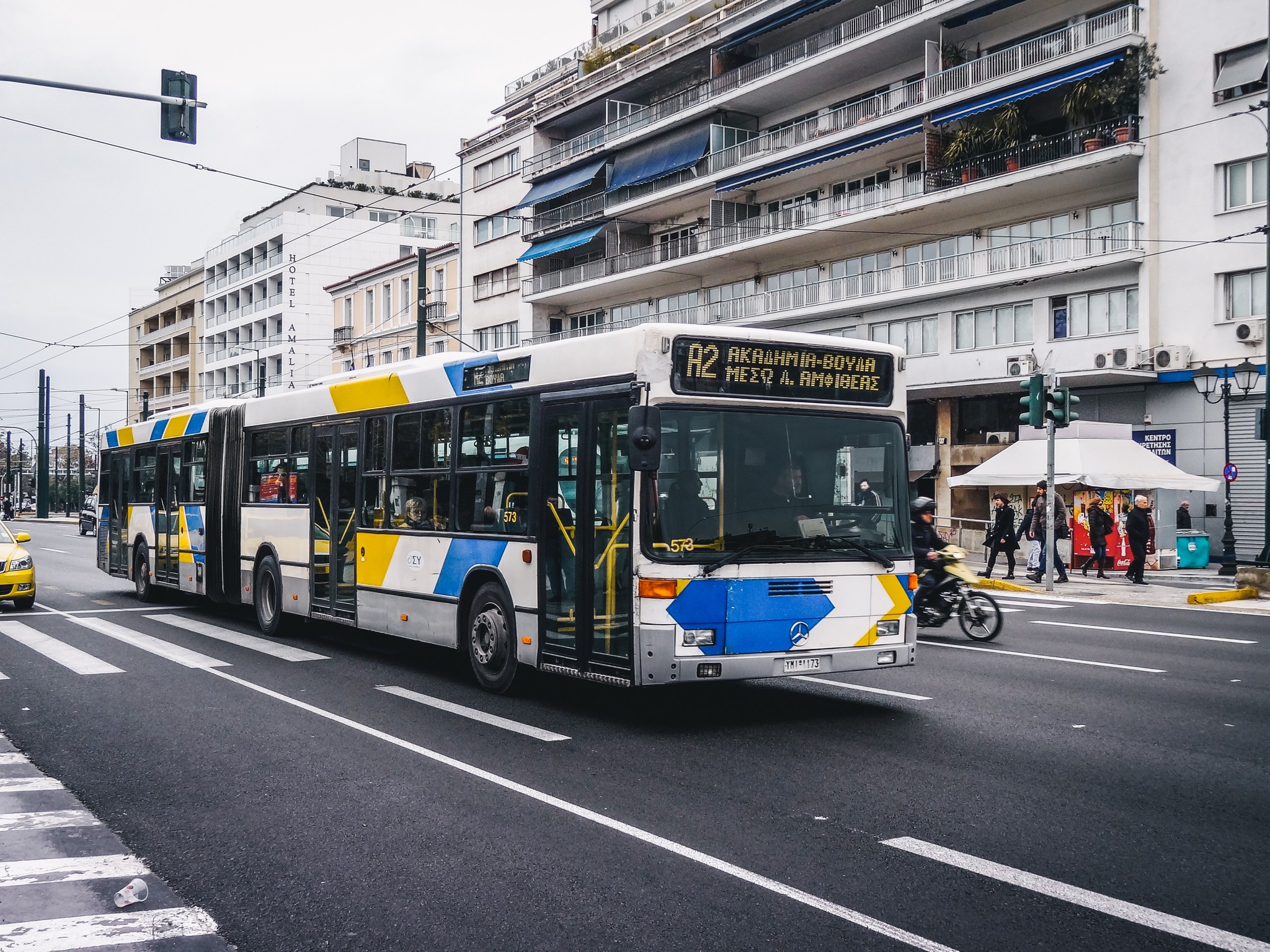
[1153,345,1190,371]
[1233,320,1264,342]
[1111,344,1141,368]
[1008,359,1034,376]
[1093,351,1113,369]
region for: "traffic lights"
[161,68,198,145]
[1044,386,1080,429]
[1019,373,1044,427]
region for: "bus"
[97,321,916,695]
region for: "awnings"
[515,156,610,207]
[516,218,614,262]
[602,113,716,193]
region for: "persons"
[755,458,824,537]
[272,462,307,504]
[979,493,1021,579]
[1081,498,1114,579]
[61,503,65,509]
[855,479,883,531]
[663,470,708,548]
[1025,479,1069,584]
[911,496,959,616]
[1176,500,1192,529]
[1125,494,1151,585]
[326,446,578,604]
[3,498,12,521]
[1034,494,1055,568]
[1016,499,1043,573]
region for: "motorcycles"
[915,544,1004,642]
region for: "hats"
[1037,480,1047,489]
[994,493,1008,499]
[1032,494,1040,507]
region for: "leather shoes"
[1125,574,1136,582]
[1133,577,1149,585]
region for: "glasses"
[409,510,428,517]
[1183,504,1190,506]
[921,512,932,514]
[275,472,287,478]
[1097,503,1102,506]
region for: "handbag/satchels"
[985,529,993,540]
[1057,524,1071,539]
[1104,521,1113,536]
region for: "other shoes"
[914,611,931,625]
[548,593,568,603]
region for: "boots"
[977,566,993,579]
[1097,558,1110,579]
[1080,556,1094,577]
[1002,570,1015,580]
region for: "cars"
[0,518,37,609]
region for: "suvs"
[78,497,98,538]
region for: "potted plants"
[938,70,1137,182]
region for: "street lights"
[0,343,265,520]
[1194,358,1260,575]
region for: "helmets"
[911,497,937,513]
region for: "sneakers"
[1054,573,1069,583]
[1026,572,1042,583]
[1026,567,1039,573]
